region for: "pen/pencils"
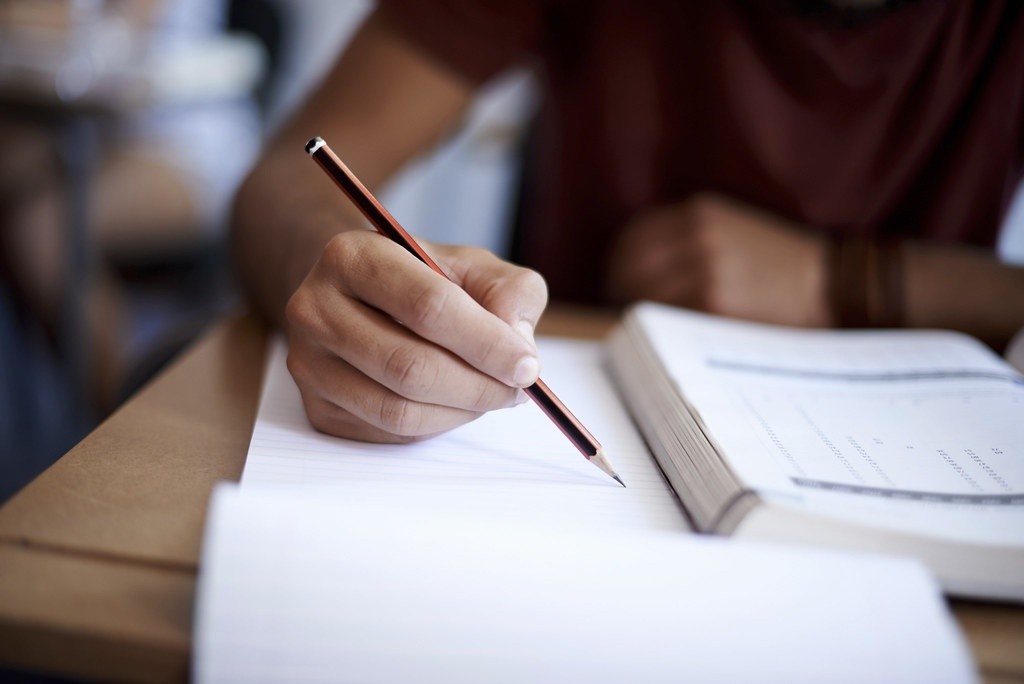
[305,134,627,488]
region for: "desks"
[0,298,1024,684]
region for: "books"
[194,331,982,684]
[606,300,1024,603]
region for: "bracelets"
[831,232,907,329]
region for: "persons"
[219,0,1024,443]
